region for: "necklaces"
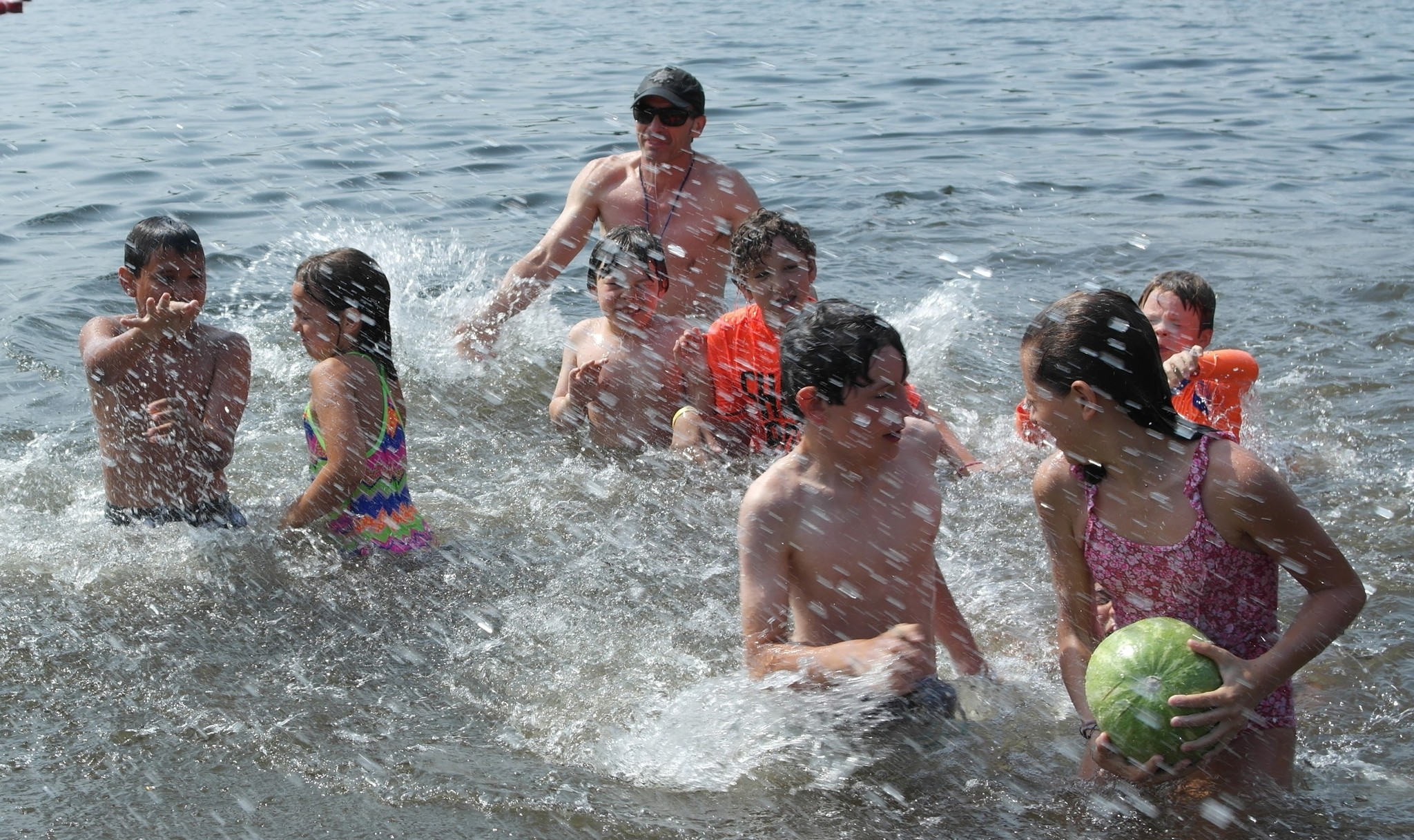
[636,149,696,239]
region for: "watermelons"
[1085,617,1224,770]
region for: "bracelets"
[673,407,698,430]
[958,461,983,476]
[1080,720,1101,739]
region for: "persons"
[1021,289,1367,791]
[80,215,251,530]
[1016,270,1258,446]
[449,65,981,478]
[736,297,989,727]
[277,248,442,566]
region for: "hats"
[630,65,705,116]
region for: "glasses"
[633,104,700,126]
